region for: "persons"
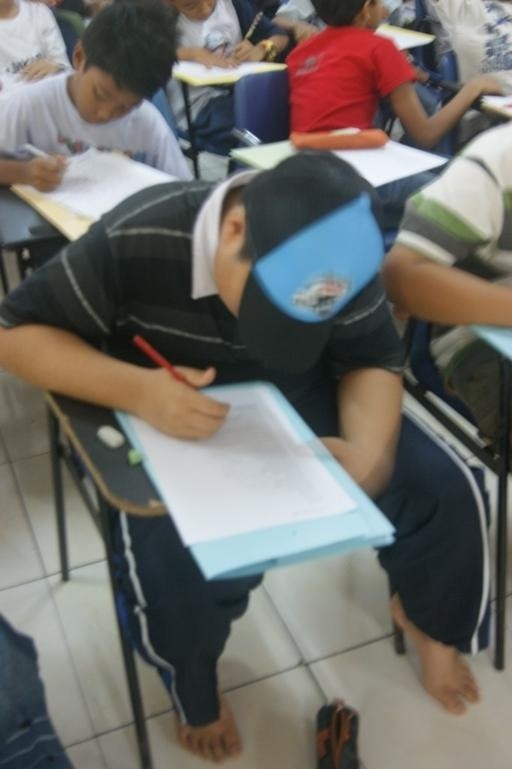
[0,0,512,195]
[383,120,512,471]
[0,150,493,766]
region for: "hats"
[236,148,385,373]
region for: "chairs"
[2,0,508,767]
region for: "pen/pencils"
[244,12,263,41]
[133,336,196,390]
[25,144,53,159]
